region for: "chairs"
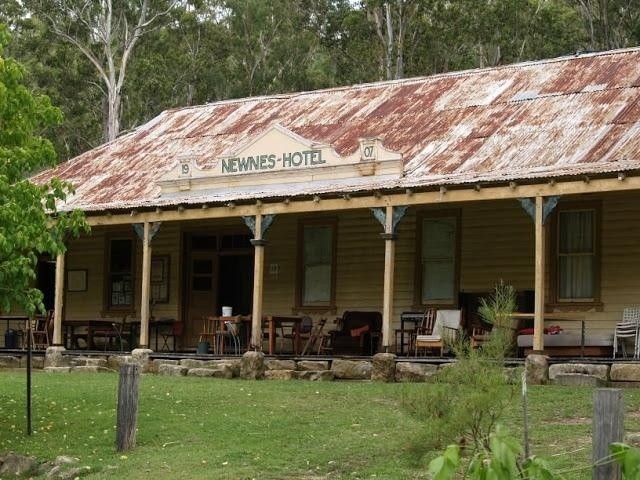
[394,308,463,358]
[198,313,333,357]
[27,310,183,355]
[612,306,639,359]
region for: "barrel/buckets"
[222,306,233,318]
[196,341,209,354]
